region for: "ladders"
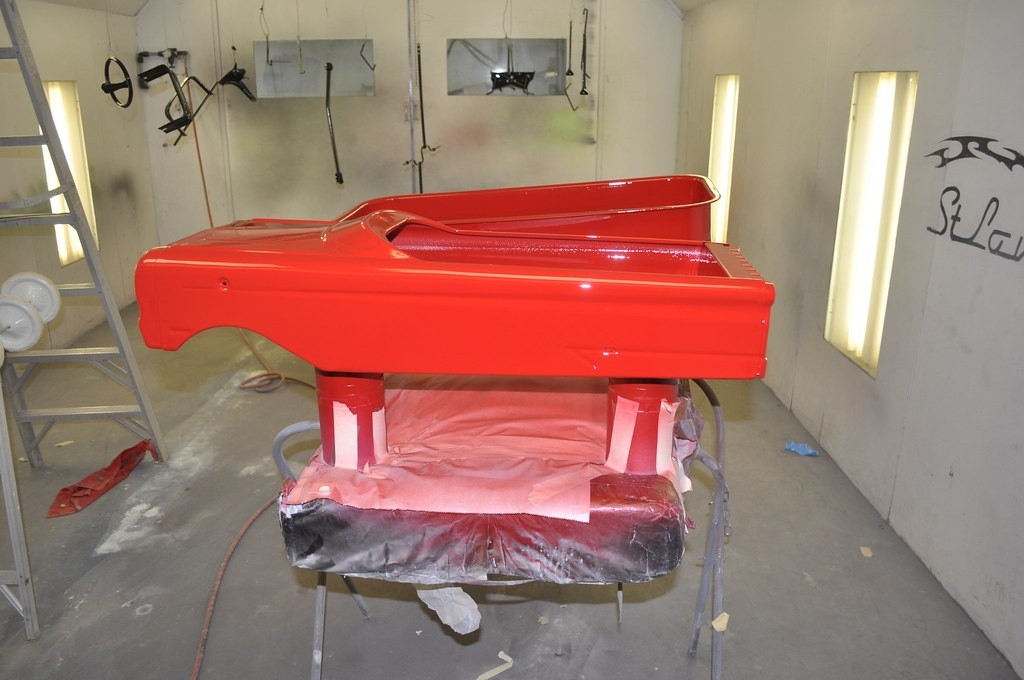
[0,1,173,644]
